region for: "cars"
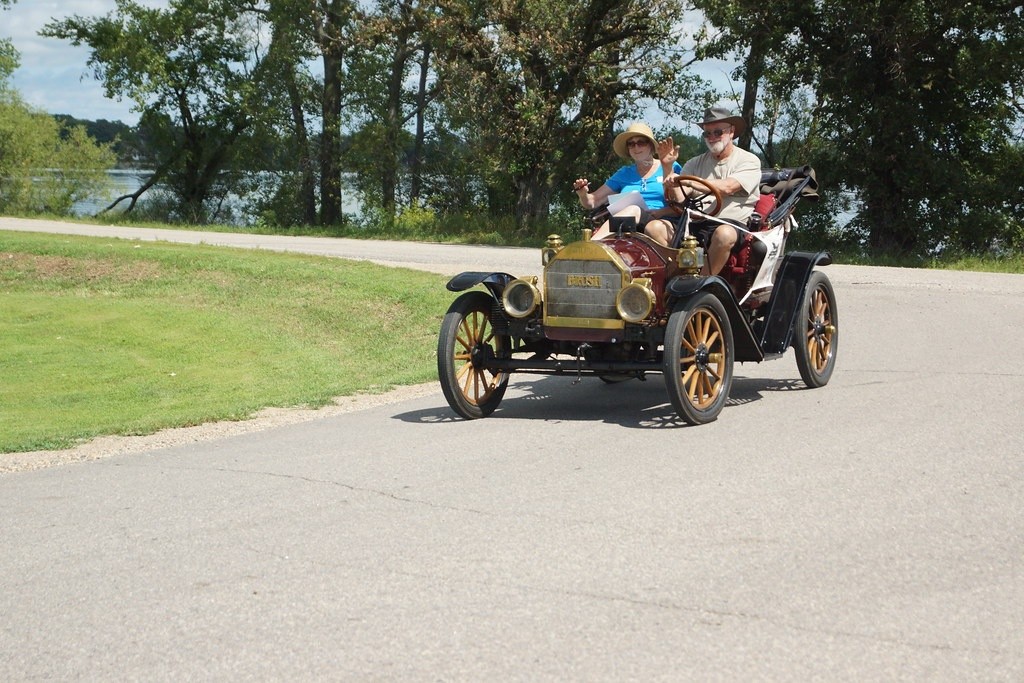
[437,165,839,424]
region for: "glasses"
[625,139,651,149]
[702,125,732,138]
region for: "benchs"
[717,193,776,275]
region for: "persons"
[644,108,762,276]
[573,124,682,241]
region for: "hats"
[612,122,660,159]
[695,107,746,140]
